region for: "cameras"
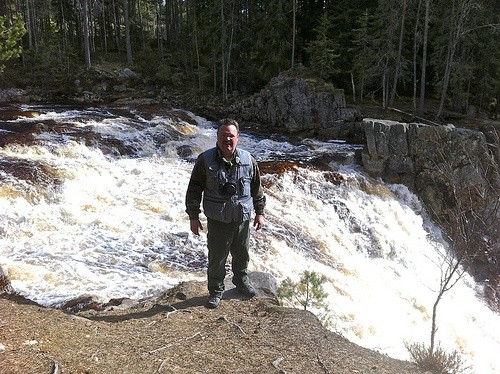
[223,182,236,196]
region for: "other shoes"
[236,280,256,297]
[207,294,221,308]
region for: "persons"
[184,118,266,308]
